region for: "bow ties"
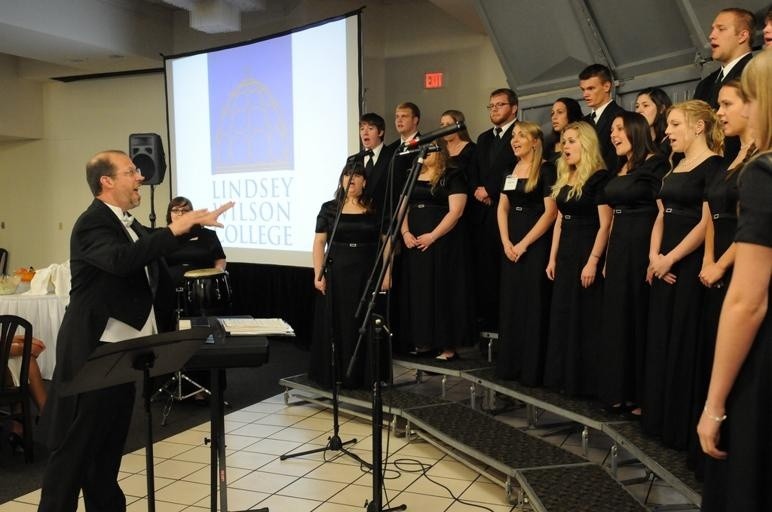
[121,214,135,228]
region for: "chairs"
[1,314,33,464]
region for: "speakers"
[129,133,166,185]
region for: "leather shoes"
[436,351,456,362]
[415,344,431,352]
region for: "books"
[218,316,296,338]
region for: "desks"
[1,291,68,388]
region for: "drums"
[184,268,233,313]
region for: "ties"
[494,127,502,141]
[716,69,724,86]
[366,151,375,168]
[590,111,597,124]
[401,142,406,152]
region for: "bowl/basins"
[0,276,22,295]
[15,271,36,282]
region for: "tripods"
[280,162,374,470]
[149,289,231,427]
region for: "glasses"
[487,102,510,110]
[171,207,192,214]
[104,168,142,179]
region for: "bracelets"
[430,233,435,242]
[400,230,410,235]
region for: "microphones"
[348,150,372,162]
[405,121,465,146]
[395,145,440,156]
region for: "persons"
[0,335,48,456]
[386,101,424,328]
[347,112,395,216]
[473,0,772,512]
[35,149,235,512]
[439,110,477,332]
[313,160,400,387]
[154,197,234,407]
[396,138,469,362]
